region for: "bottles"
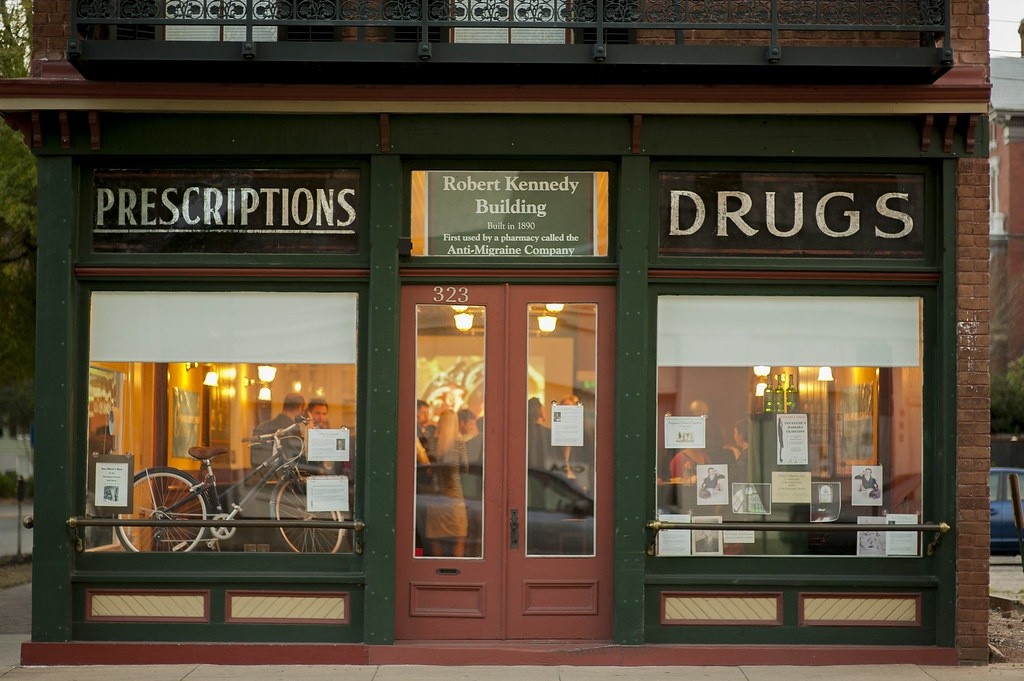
[774,375,784,413]
[763,374,774,414]
[786,375,799,414]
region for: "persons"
[336,440,345,451]
[252,387,749,557]
[696,467,725,498]
[777,419,784,462]
[853,468,879,496]
[695,531,718,552]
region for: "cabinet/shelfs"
[796,367,892,521]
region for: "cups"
[244,544,256,552]
[415,548,423,556]
[257,544,271,552]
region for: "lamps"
[454,313,473,330]
[204,370,221,389]
[753,366,772,377]
[257,387,275,403]
[258,365,278,383]
[754,382,767,397]
[452,305,469,312]
[817,367,836,381]
[545,303,565,312]
[537,316,558,333]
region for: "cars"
[412,461,595,557]
[988,467,1024,553]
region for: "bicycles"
[112,409,348,552]
